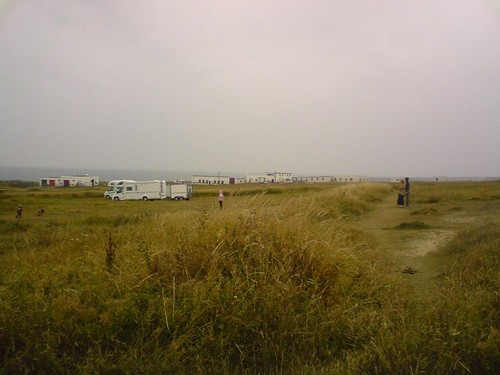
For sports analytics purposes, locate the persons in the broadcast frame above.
[404,177,410,206]
[16,204,23,219]
[218,190,223,209]
[397,179,405,208]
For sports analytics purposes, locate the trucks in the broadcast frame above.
[104,178,193,202]
